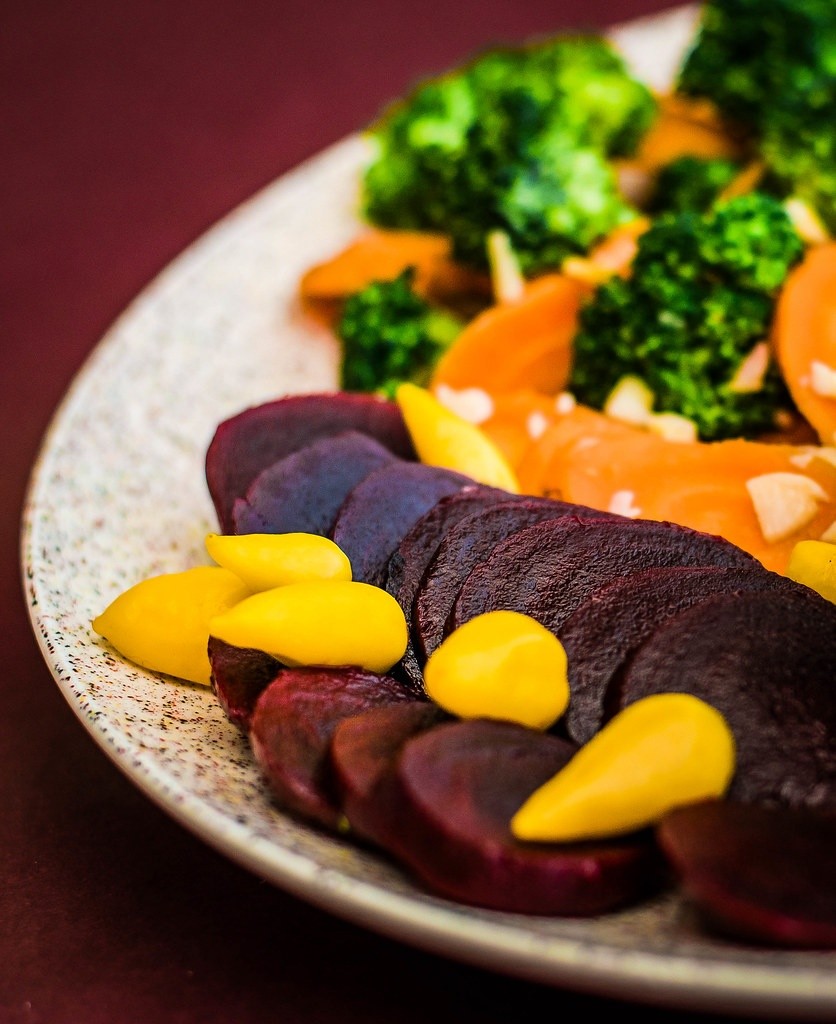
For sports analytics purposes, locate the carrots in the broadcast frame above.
[294,84,835,606]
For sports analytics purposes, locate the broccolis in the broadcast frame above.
[328,0,836,441]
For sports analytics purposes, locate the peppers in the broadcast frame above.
[91,531,736,844]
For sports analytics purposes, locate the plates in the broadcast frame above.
[20,3,836,1019]
